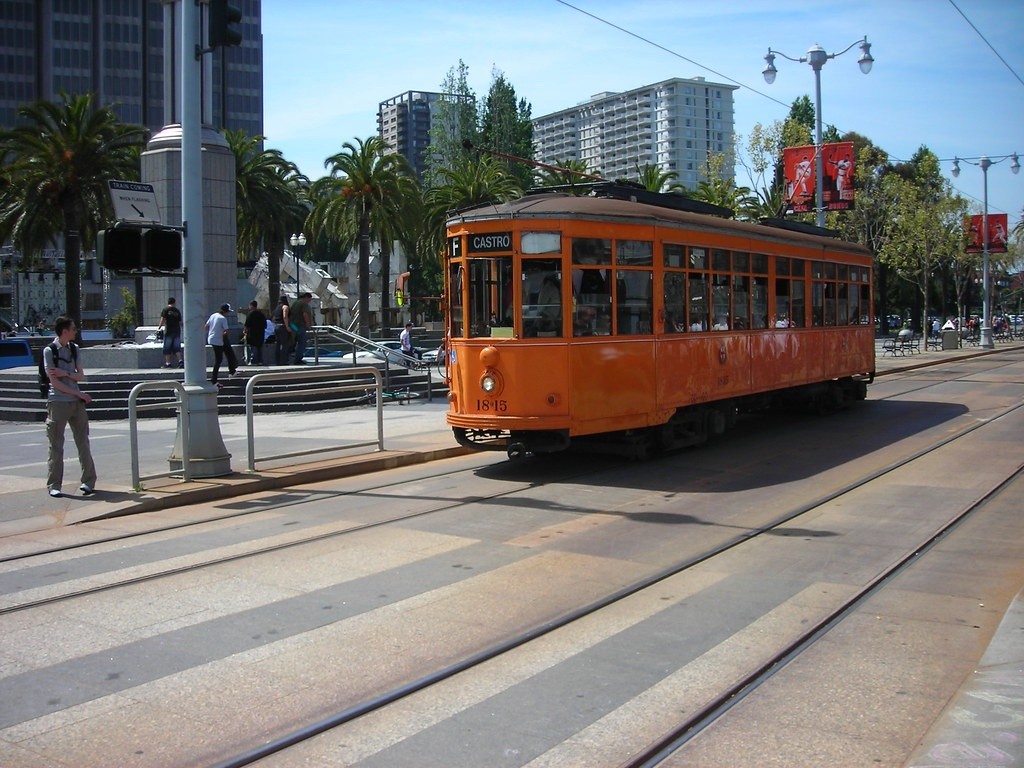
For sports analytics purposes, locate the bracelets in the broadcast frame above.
[65,370,66,377]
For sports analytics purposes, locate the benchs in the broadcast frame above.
[926,332,947,352]
[900,332,924,355]
[965,331,981,346]
[992,327,1024,343]
[882,335,906,357]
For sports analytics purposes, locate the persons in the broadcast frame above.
[400,324,423,367]
[205,303,241,389]
[39,319,47,336]
[828,154,850,198]
[243,301,267,366]
[158,297,184,369]
[992,313,1010,336]
[928,317,939,339]
[795,156,811,195]
[43,317,96,496]
[502,262,561,337]
[274,293,312,365]
[992,221,1007,244]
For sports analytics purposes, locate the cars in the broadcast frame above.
[421,347,446,364]
[302,346,345,358]
[343,340,422,366]
[969,314,1024,325]
[861,314,941,329]
[0,339,35,370]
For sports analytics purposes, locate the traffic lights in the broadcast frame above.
[207,0,243,46]
[143,228,182,272]
[93,223,143,269]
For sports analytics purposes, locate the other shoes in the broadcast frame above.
[294,360,306,364]
[49,488,61,496]
[178,360,184,368]
[212,383,223,388]
[79,482,92,495]
[161,363,171,368]
[229,371,239,378]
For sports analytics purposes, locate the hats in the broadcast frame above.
[221,303,234,312]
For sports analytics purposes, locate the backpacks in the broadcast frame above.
[39,341,77,398]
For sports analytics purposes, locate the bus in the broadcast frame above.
[436,177,876,466]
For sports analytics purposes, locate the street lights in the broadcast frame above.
[290,233,307,298]
[951,151,1022,351]
[761,32,875,223]
